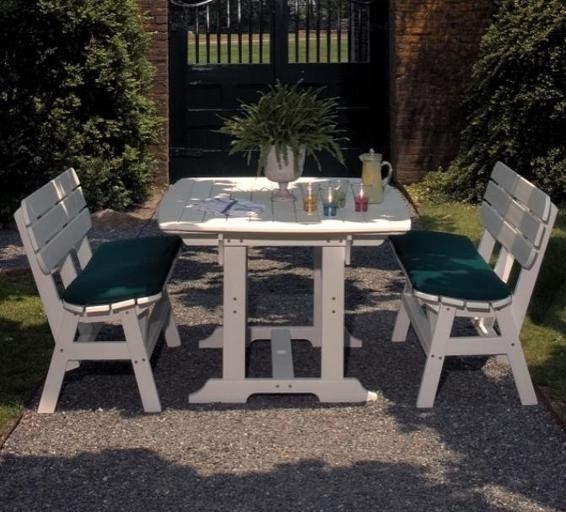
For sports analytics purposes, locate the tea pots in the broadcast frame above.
[359,148,392,204]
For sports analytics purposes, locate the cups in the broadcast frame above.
[296,181,320,217]
[319,182,337,219]
[351,182,373,214]
[331,179,349,208]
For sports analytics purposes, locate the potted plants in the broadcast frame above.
[213,74,354,205]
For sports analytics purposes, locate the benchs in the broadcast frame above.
[13,167,184,418]
[388,160,560,409]
